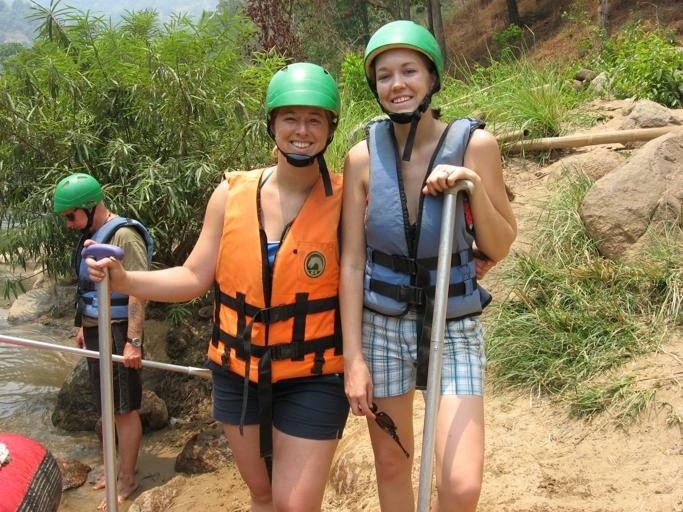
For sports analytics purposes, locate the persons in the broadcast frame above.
[53,172,154,511]
[338,20,518,512]
[83,61,495,512]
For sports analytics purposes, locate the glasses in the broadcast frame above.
[66,208,77,220]
[370,402,411,459]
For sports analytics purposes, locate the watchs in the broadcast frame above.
[127,337,143,347]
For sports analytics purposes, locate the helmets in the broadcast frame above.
[54,172,104,213]
[265,61,341,120]
[364,20,444,93]
